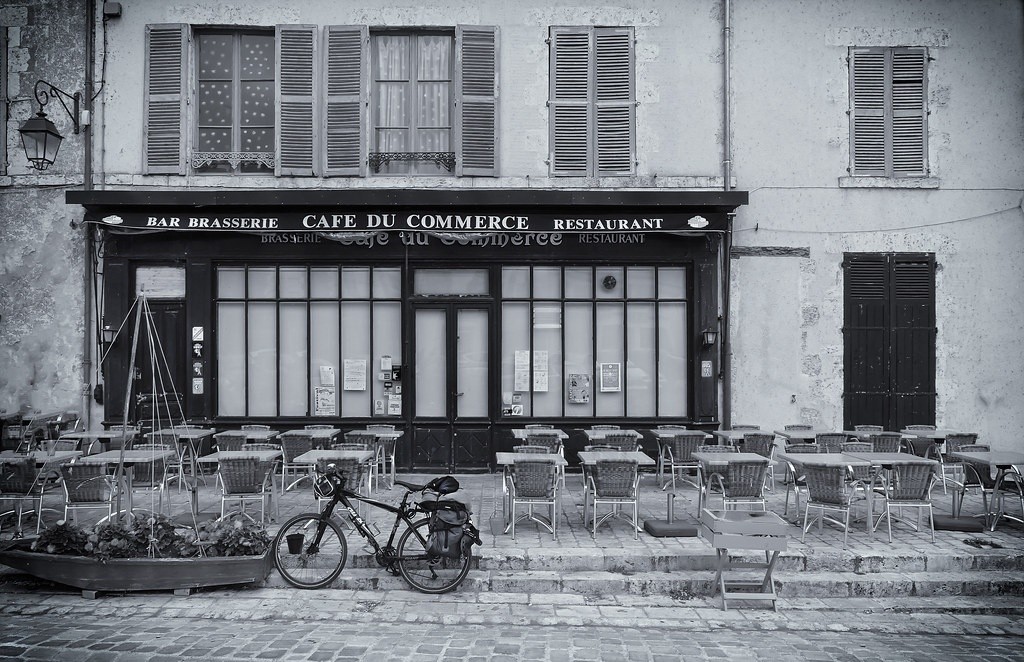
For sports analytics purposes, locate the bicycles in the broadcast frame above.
[272,457,482,596]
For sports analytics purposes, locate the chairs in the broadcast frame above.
[0,412,398,535]
[502,425,1024,550]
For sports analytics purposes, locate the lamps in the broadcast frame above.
[18,79,80,171]
[703,325,719,350]
[98,323,118,357]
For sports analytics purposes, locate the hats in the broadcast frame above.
[464,522,482,546]
[461,536,474,556]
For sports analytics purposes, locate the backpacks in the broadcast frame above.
[425,510,469,557]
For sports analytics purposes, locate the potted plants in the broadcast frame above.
[1,515,279,592]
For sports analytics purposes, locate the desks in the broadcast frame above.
[0,449,84,530]
[583,430,644,440]
[144,429,217,492]
[776,452,871,536]
[213,430,279,439]
[712,431,776,445]
[62,431,140,491]
[649,429,714,489]
[276,429,341,491]
[951,451,1024,529]
[80,449,176,531]
[197,451,282,526]
[577,452,655,533]
[700,508,790,613]
[495,452,568,533]
[841,452,939,529]
[343,430,405,489]
[900,430,978,440]
[691,453,778,511]
[841,431,902,437]
[774,430,834,482]
[293,450,374,530]
[510,429,569,439]
